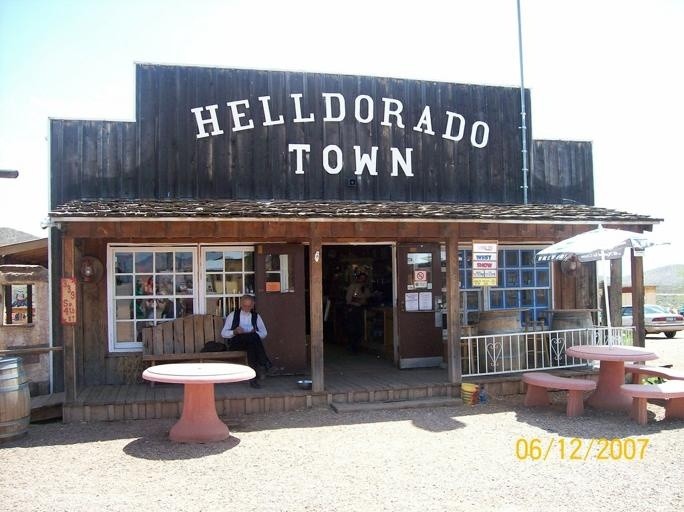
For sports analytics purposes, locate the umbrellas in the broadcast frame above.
[535,222,674,349]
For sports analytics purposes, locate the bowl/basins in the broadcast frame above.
[296,380,313,390]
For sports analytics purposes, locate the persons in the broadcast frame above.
[219,294,280,388]
[143,275,152,322]
[342,271,370,356]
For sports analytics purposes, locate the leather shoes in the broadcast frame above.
[250,379,262,390]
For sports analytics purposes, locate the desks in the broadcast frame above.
[142,362,257,443]
[566,345,659,409]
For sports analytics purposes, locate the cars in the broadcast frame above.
[621,304,684,338]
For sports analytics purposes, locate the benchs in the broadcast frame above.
[141,314,249,388]
[521,363,684,425]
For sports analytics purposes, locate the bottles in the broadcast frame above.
[480,386,488,406]
